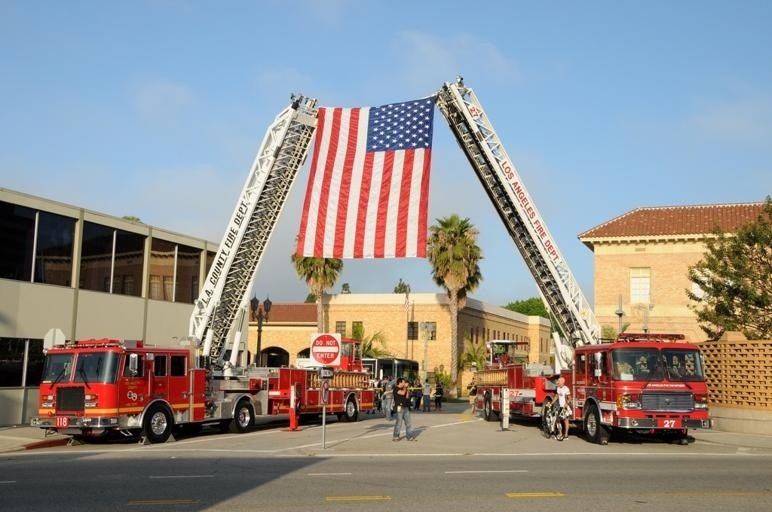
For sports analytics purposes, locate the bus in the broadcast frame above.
[362,359,420,412]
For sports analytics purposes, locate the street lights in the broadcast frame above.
[249,294,272,367]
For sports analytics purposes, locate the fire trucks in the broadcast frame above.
[32,94,378,445]
[436,76,713,445]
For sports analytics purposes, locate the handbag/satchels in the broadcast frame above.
[401,398,414,407]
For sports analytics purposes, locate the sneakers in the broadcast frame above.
[407,436,418,441]
[563,435,569,440]
[391,436,402,441]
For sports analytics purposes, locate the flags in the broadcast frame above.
[296,96,436,259]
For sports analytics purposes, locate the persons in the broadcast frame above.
[546,377,572,441]
[467,382,477,417]
[392,378,415,442]
[372,376,443,421]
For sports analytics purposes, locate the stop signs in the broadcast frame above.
[309,333,342,367]
